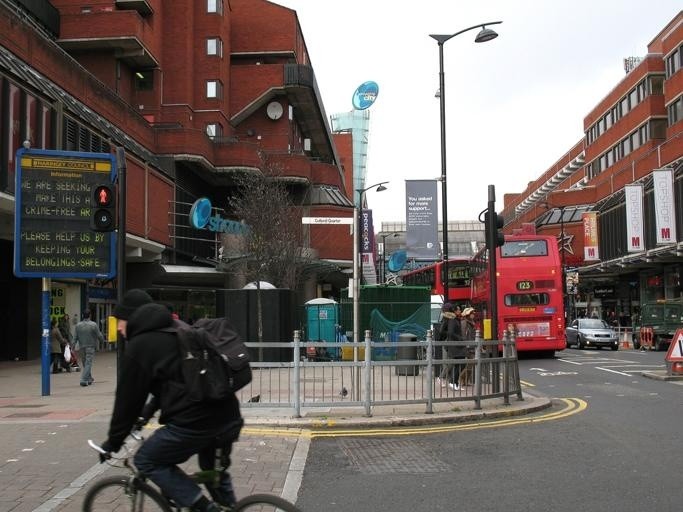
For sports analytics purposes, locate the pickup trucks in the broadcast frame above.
[631,298,682,352]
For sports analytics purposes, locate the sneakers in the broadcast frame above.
[204,501,224,512]
[436,377,446,388]
[458,386,464,391]
[449,382,458,391]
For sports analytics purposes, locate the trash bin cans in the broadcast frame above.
[395,333,419,376]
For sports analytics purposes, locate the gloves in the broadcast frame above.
[134,418,144,430]
[99,438,121,463]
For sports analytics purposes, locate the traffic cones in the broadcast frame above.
[620,329,630,349]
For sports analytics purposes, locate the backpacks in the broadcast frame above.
[155,317,251,402]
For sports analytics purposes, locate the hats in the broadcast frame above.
[111,288,155,322]
[461,308,474,316]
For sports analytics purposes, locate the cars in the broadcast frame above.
[565,318,619,352]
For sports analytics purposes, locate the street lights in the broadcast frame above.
[536,201,569,310]
[426,19,504,307]
[355,181,390,287]
[380,232,406,282]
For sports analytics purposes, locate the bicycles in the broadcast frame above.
[79,418,299,512]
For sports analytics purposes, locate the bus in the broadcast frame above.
[401,257,486,310]
[469,234,566,359]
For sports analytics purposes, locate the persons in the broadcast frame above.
[99,286,245,512]
[50,313,79,373]
[138,300,238,509]
[71,308,104,387]
[436,302,477,392]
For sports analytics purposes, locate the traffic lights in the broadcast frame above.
[88,181,116,234]
[485,210,504,249]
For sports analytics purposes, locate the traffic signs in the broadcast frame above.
[12,145,117,281]
